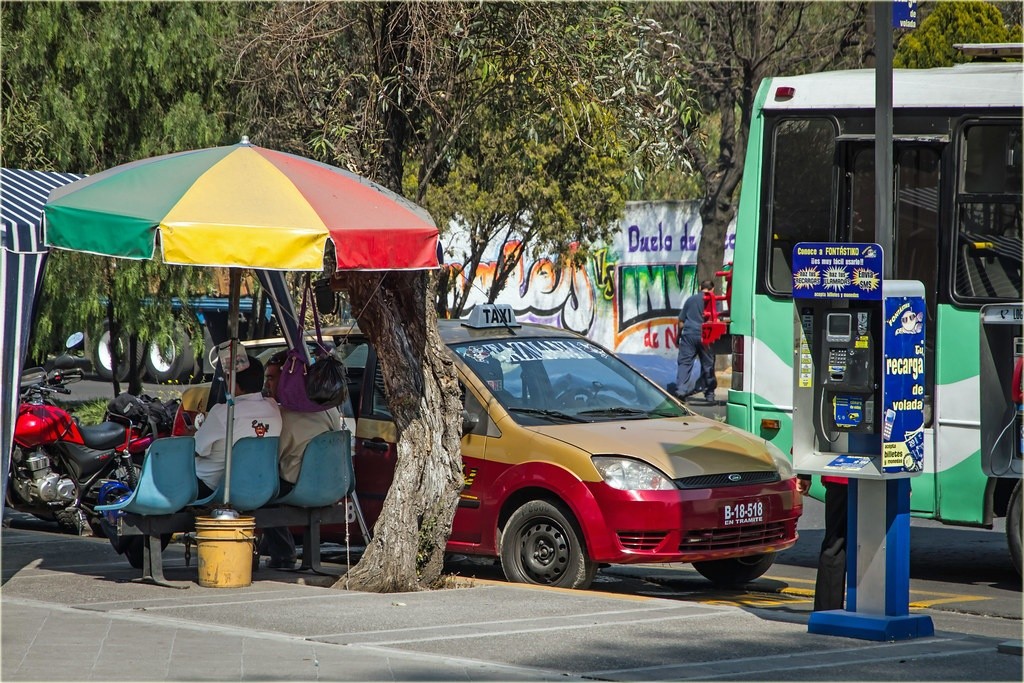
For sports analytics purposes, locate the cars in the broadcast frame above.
[215,302,806,591]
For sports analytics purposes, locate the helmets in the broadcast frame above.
[98,481,133,518]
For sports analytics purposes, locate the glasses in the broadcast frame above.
[267,355,285,366]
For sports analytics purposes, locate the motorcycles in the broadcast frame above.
[6,330,192,542]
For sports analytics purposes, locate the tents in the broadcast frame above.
[0,167,87,513]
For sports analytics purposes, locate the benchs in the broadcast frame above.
[95,430,372,589]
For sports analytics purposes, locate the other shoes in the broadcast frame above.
[704,392,715,401]
[124,547,138,568]
[101,518,123,555]
[252,558,259,571]
[265,554,297,568]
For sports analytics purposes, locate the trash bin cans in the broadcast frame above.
[193,514,256,590]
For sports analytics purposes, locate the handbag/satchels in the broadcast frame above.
[277,346,348,412]
[107,394,181,433]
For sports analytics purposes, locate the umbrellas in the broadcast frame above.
[39,137,442,508]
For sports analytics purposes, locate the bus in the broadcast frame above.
[727,41,1023,587]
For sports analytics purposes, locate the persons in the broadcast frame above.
[99,352,358,572]
[675,280,719,403]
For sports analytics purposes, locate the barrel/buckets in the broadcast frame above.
[195,514,258,589]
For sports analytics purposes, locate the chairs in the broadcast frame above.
[468,355,515,415]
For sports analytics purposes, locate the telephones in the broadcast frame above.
[819,308,875,394]
